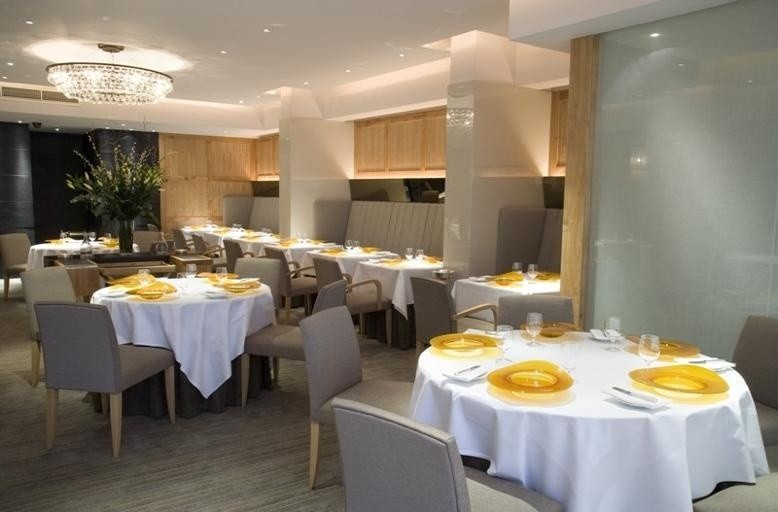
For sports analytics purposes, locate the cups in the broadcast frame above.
[559,340,582,374]
[636,333,664,365]
[232,224,242,233]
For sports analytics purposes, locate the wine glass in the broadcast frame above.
[49,230,119,247]
[525,311,543,348]
[511,261,539,284]
[493,324,514,363]
[601,317,621,353]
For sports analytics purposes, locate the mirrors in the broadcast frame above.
[541,176,564,208]
[250,181,279,197]
[348,178,445,205]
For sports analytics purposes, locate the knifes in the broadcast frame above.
[612,384,657,404]
[454,364,480,375]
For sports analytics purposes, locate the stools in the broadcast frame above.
[692,468,778,511]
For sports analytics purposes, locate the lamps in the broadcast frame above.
[44,43,176,107]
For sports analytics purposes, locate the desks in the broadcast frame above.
[183,223,442,353]
[90,273,272,425]
[29,240,138,267]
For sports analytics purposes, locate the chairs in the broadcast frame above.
[297,304,413,489]
[20,268,77,385]
[329,397,566,511]
[239,279,348,411]
[32,301,176,457]
[131,223,254,273]
[233,258,282,326]
[1,233,33,297]
[410,276,499,361]
[730,313,777,448]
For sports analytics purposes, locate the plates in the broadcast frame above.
[475,371,491,381]
[616,397,644,408]
[470,277,486,282]
[485,362,574,396]
[277,237,323,248]
[712,367,729,373]
[428,332,496,355]
[626,364,730,397]
[318,240,438,267]
[519,319,578,337]
[487,269,557,287]
[626,330,700,359]
[588,333,595,340]
[107,264,265,300]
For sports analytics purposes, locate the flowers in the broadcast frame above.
[63,129,179,255]
